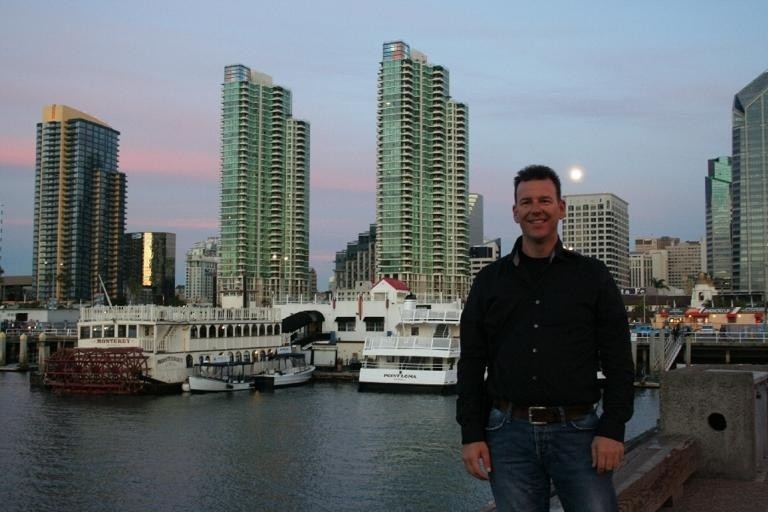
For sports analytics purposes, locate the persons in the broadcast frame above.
[455,165,634,511]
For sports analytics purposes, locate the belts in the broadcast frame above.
[494,399,595,425]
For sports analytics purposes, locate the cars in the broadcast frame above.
[1,303,66,309]
[628,323,719,339]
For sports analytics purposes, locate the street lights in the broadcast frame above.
[270,252,290,304]
[42,260,63,309]
[640,291,647,323]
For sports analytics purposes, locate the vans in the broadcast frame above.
[719,322,767,343]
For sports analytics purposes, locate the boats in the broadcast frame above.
[632,379,659,389]
